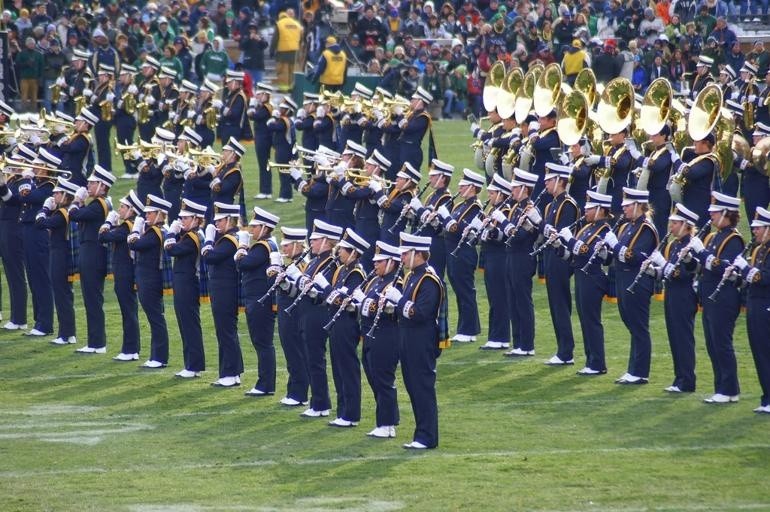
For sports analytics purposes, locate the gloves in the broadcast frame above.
[411,196,421,210]
[158,158,164,166]
[272,107,324,118]
[289,168,301,181]
[286,263,330,289]
[470,121,544,145]
[559,153,569,164]
[401,204,416,217]
[270,251,284,266]
[354,101,402,118]
[55,77,156,105]
[106,210,118,225]
[133,150,142,159]
[437,205,505,231]
[238,231,249,250]
[370,182,383,193]
[630,146,641,159]
[205,224,217,243]
[177,162,188,171]
[338,286,403,304]
[43,197,57,211]
[209,165,216,179]
[518,207,617,260]
[187,110,195,119]
[641,237,747,281]
[583,155,601,167]
[148,95,179,124]
[73,188,87,201]
[671,152,679,163]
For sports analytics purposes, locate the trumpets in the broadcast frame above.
[320,84,411,121]
[114,136,224,167]
[266,141,397,189]
[1,107,75,180]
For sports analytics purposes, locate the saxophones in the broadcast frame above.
[48,64,230,129]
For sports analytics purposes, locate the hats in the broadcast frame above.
[429,158,570,197]
[683,56,770,136]
[279,220,431,262]
[316,140,422,183]
[583,187,770,229]
[0,101,118,193]
[178,69,296,110]
[119,188,278,231]
[72,49,177,82]
[302,81,434,106]
[151,124,245,157]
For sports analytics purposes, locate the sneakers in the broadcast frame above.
[254,194,273,199]
[451,334,606,375]
[617,372,770,413]
[276,196,293,203]
[3,320,139,361]
[142,361,275,396]
[281,396,428,449]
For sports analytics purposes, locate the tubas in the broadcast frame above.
[470,59,770,183]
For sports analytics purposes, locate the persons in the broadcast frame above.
[1,0,770,120]
[1,54,452,451]
[447,109,770,412]
